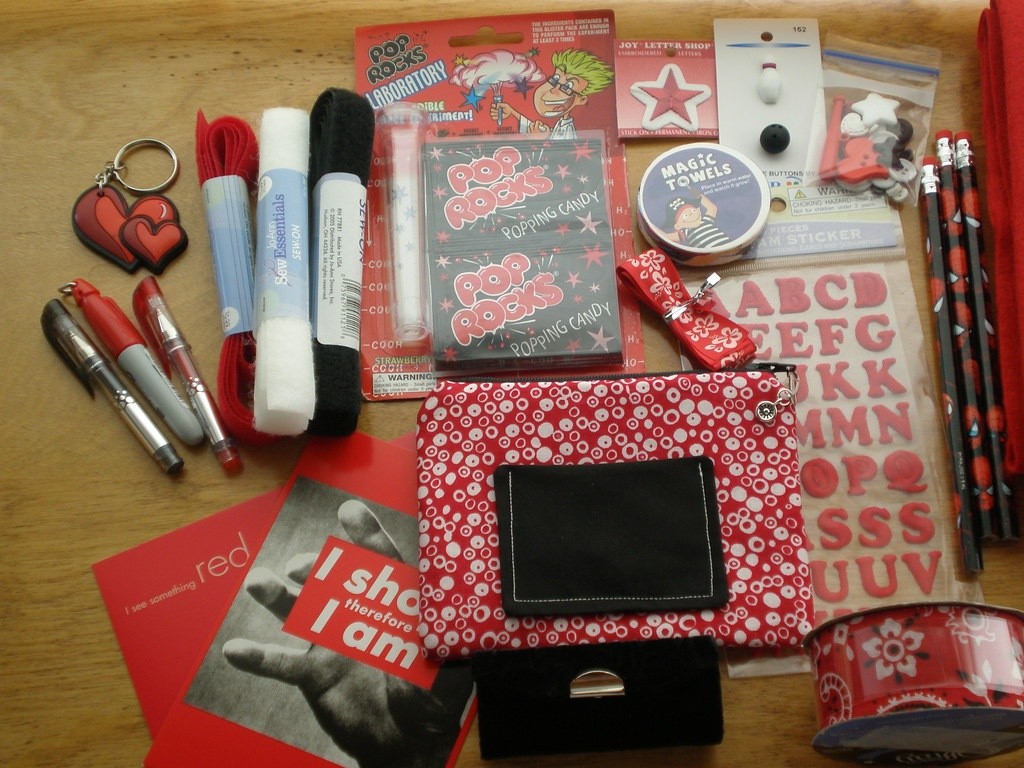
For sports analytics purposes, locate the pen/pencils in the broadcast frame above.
[132,274,243,476]
[920,126,1023,574]
[40,298,185,475]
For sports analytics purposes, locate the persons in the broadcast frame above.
[223,499,478,768]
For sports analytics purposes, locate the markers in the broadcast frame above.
[54,279,206,447]
